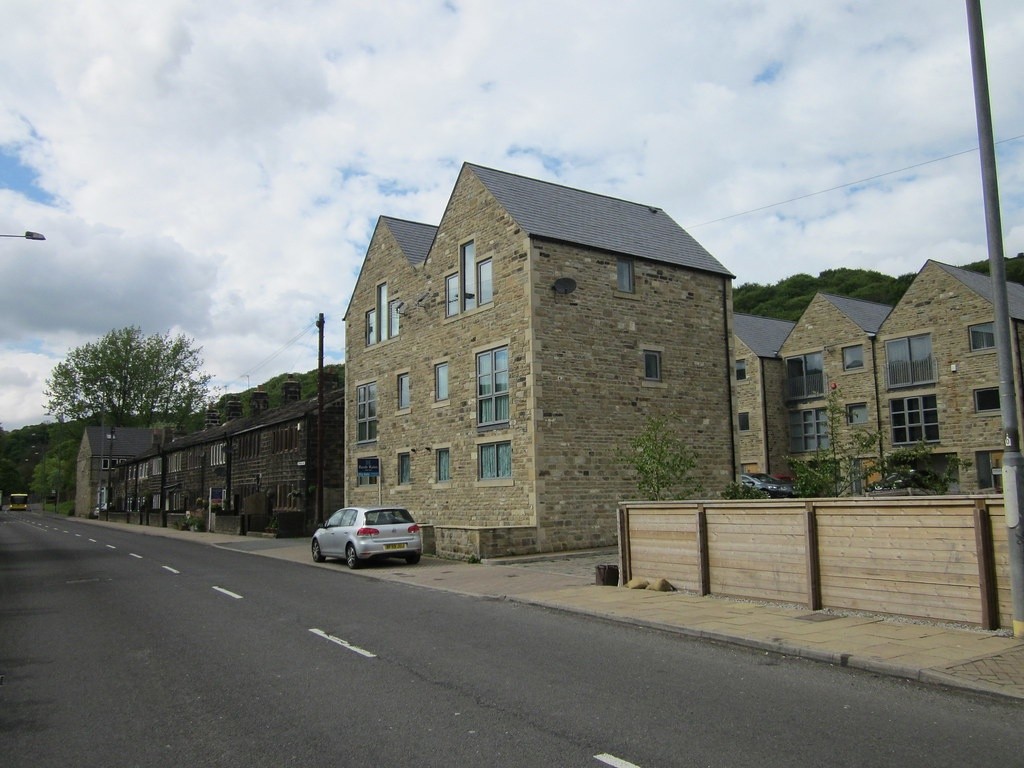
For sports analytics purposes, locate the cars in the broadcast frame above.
[864,469,939,493]
[741,473,800,499]
[93,501,113,518]
[311,506,422,570]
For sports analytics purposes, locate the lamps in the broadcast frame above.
[395,302,407,319]
[416,294,427,309]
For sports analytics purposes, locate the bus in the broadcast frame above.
[8,494,28,511]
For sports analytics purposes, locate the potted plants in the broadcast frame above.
[265,516,279,533]
[182,514,207,533]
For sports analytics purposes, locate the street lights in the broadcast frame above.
[32,432,45,510]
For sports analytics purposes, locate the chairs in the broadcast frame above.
[375,512,389,525]
